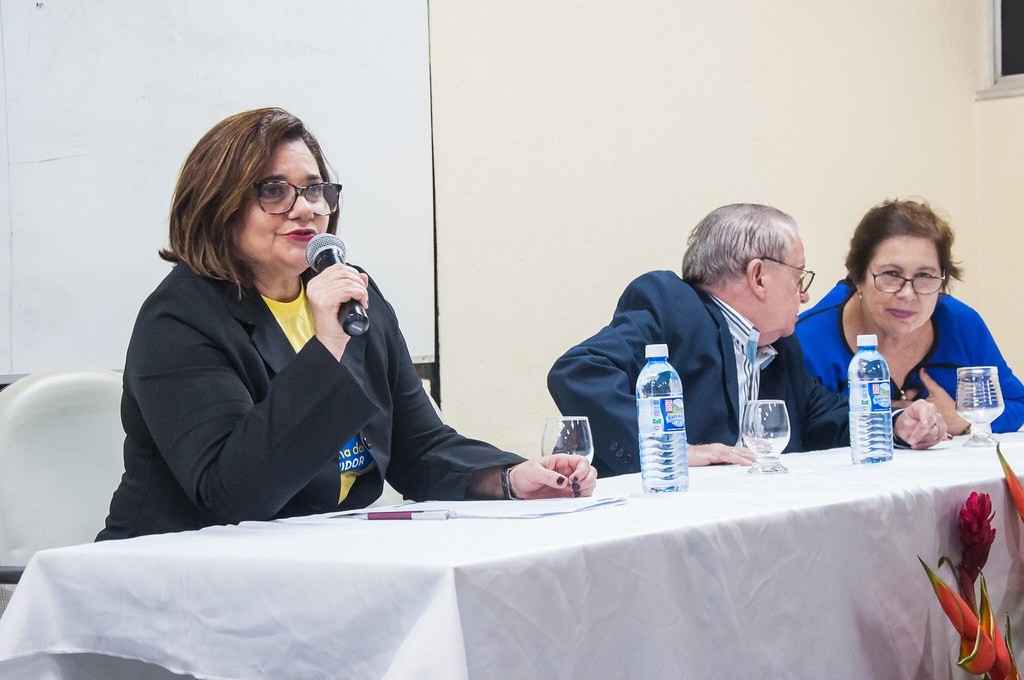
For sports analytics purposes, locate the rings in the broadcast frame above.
[731,449,738,454]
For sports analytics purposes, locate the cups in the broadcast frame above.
[540,415,594,467]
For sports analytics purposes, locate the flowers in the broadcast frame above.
[914,442,1024,680]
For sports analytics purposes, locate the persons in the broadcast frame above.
[547,203,947,481]
[94,107,597,542]
[794,197,1024,436]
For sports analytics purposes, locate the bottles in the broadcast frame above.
[847,335,894,465]
[635,343,690,497]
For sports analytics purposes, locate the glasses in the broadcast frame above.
[253,180,342,216]
[761,256,815,292]
[865,264,946,295]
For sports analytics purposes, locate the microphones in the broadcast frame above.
[304,234,371,337]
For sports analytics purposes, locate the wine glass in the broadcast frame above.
[742,399,791,475]
[954,366,1005,448]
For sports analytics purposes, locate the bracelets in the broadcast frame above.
[501,464,521,500]
[959,423,971,436]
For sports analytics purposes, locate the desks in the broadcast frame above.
[0,432,1024,680]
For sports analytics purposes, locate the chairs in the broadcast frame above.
[0,371,129,604]
